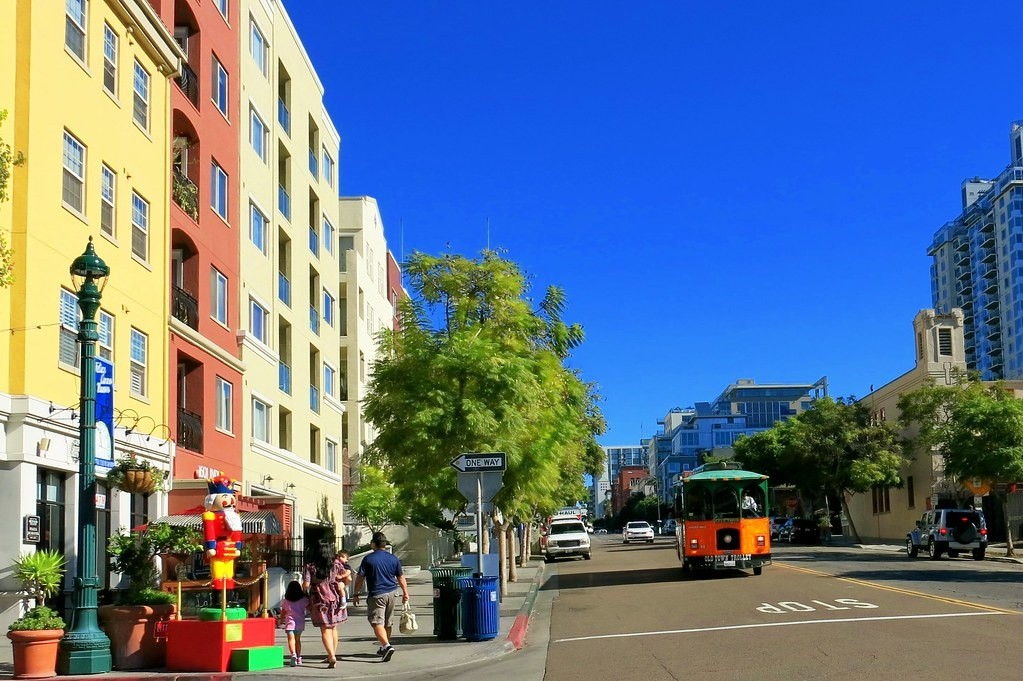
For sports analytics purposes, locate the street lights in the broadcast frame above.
[56,233,112,676]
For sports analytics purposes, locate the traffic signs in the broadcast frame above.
[450,451,507,473]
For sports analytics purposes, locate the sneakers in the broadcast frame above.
[376,646,384,656]
[297,657,303,666]
[382,645,394,662]
[290,654,297,666]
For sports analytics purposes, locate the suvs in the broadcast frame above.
[905,508,988,561]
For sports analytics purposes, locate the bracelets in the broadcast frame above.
[353,594,359,597]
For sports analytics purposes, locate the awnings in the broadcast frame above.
[154,511,281,535]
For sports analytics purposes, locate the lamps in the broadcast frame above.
[264,474,274,483]
[286,482,296,489]
[49,402,171,446]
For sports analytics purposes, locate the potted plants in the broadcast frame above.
[6,548,71,679]
[98,522,204,670]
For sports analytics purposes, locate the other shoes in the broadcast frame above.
[338,602,347,609]
[328,657,336,668]
[322,658,330,663]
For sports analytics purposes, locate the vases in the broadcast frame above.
[117,469,157,493]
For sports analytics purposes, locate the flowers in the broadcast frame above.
[105,450,166,498]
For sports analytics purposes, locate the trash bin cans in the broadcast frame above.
[429,566,474,641]
[458,576,499,642]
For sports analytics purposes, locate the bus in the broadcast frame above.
[671,461,773,575]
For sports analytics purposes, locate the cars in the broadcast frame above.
[622,520,659,544]
[661,518,676,536]
[542,520,592,564]
[769,515,791,542]
[778,516,826,547]
[543,505,621,534]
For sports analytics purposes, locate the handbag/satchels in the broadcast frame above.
[399,601,418,634]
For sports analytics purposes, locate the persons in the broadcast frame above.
[736,488,758,511]
[278,581,311,666]
[336,549,351,610]
[302,539,352,669]
[203,476,243,590]
[353,532,409,663]
